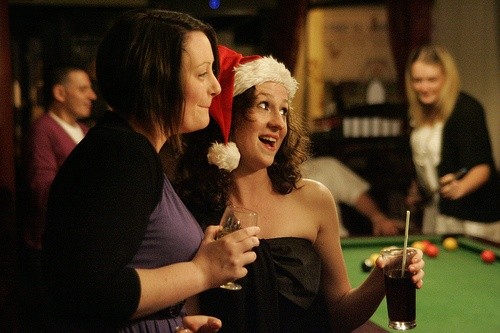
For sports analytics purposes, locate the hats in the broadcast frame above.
[202,43,299,174]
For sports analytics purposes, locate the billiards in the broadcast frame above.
[480,249,496,263]
[411,240,424,254]
[362,258,375,272]
[369,253,380,266]
[422,239,432,253]
[442,237,459,252]
[426,244,440,257]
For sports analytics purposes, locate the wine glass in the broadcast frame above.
[218,204,257,289]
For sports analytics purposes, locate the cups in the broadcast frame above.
[381,247,418,330]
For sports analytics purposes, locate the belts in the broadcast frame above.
[138,298,188,319]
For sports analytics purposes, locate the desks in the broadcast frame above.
[341,232,500,333]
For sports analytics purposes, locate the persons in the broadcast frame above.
[389,45,500,244]
[23,62,97,251]
[297,156,409,240]
[29,8,261,332]
[159,45,421,332]
